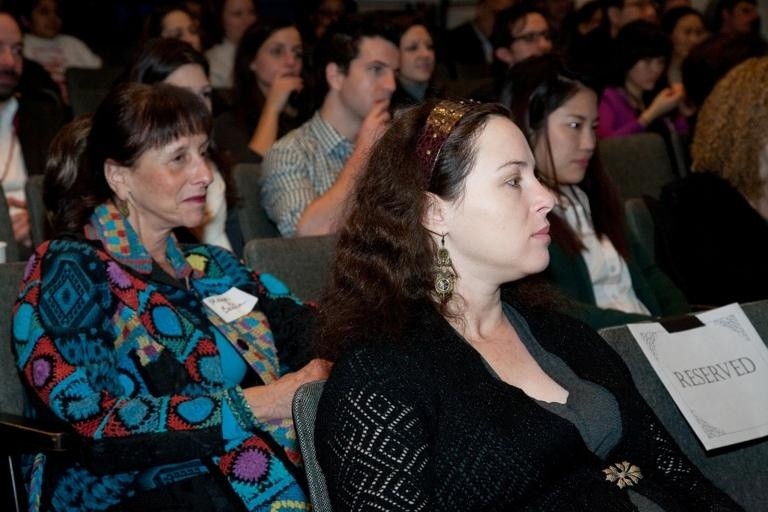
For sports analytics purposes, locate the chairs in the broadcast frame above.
[0,52,766,512]
